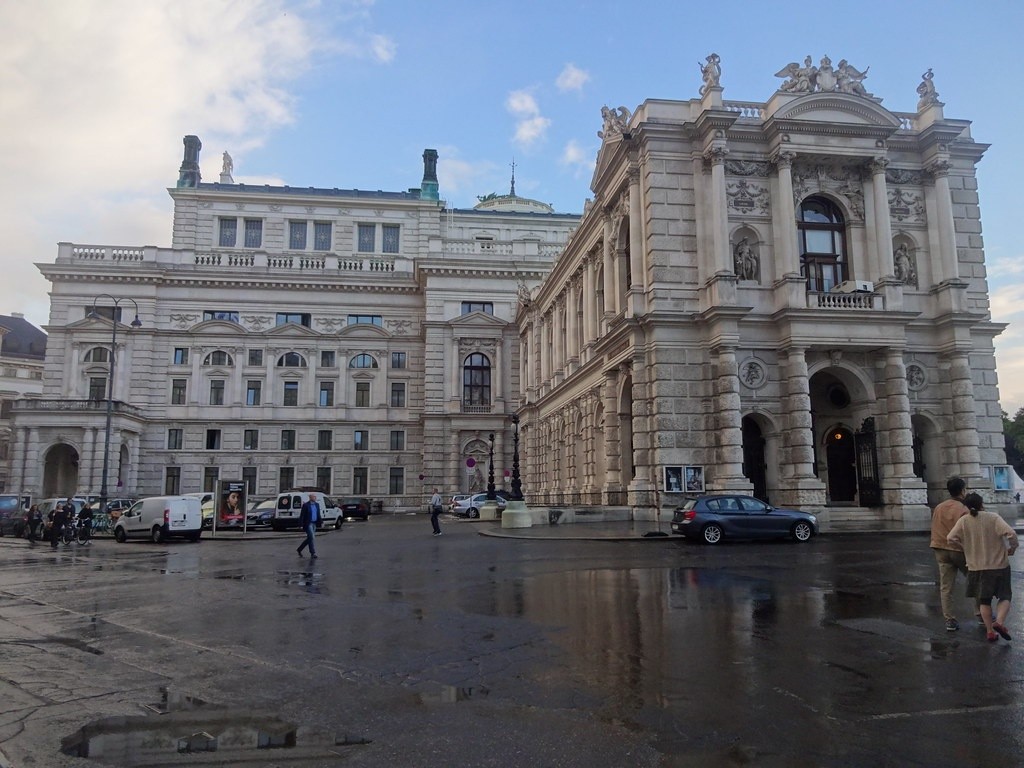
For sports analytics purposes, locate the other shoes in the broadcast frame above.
[987,633,999,641]
[311,555,317,558]
[51,542,59,547]
[946,620,959,631]
[993,622,1011,641]
[297,550,303,556]
[88,540,92,544]
[432,531,441,536]
[978,614,997,625]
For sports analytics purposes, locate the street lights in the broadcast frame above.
[87,293,143,515]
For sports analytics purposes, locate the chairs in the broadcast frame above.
[720,500,729,509]
[731,501,738,509]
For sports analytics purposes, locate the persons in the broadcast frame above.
[27,505,42,543]
[947,494,1019,642]
[832,59,883,105]
[735,236,758,281]
[78,503,93,545]
[220,491,242,525]
[469,466,484,490]
[895,243,915,285]
[296,493,323,558]
[48,503,64,547]
[595,105,632,138]
[63,498,76,533]
[697,52,722,94]
[222,149,233,173]
[776,55,818,93]
[929,476,995,630]
[918,66,943,109]
[427,488,442,536]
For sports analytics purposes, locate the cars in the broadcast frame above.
[453,494,508,518]
[25,498,90,541]
[338,497,372,521]
[0,496,28,537]
[673,492,819,544]
[246,498,276,527]
[91,498,137,519]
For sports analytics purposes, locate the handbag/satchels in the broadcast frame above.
[47,521,53,528]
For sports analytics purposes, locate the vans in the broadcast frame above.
[275,490,344,531]
[113,496,204,544]
[449,495,471,515]
[182,493,214,528]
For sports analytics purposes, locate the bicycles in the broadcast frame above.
[92,515,117,535]
[61,517,92,545]
